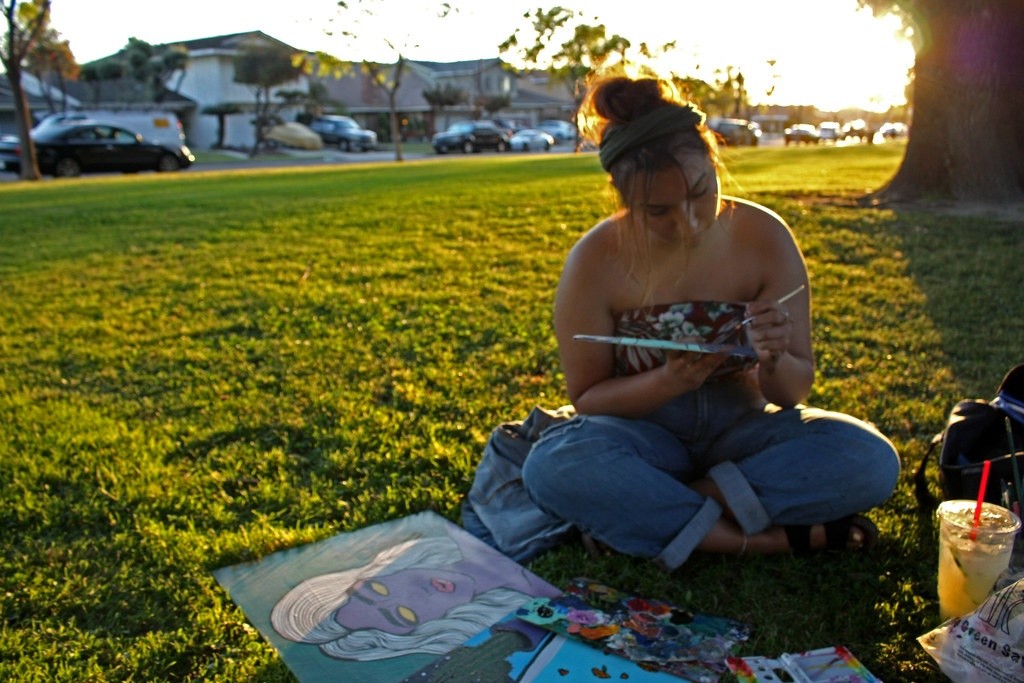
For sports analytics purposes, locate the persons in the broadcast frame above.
[521,75,901,573]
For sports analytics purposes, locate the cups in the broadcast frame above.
[936,498,1022,626]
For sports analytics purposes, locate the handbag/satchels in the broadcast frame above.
[915,578,1024,683]
[909,395,1023,535]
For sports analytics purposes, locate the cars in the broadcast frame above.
[311,115,378,153]
[498,119,531,132]
[0,120,194,180]
[714,120,760,146]
[784,124,821,146]
[507,129,555,152]
[431,120,511,155]
[538,120,576,141]
[818,119,910,144]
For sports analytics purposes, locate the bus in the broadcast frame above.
[32,110,187,153]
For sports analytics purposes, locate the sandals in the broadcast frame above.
[581,531,611,560]
[780,513,881,558]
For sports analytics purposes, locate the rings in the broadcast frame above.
[783,311,789,321]
